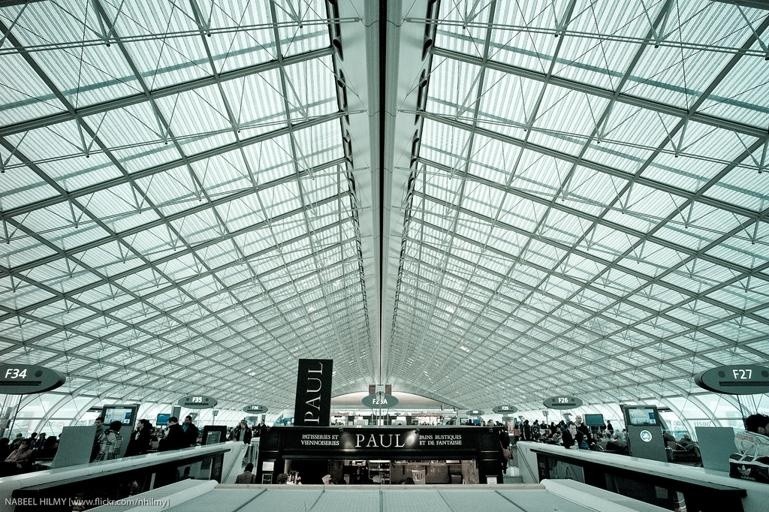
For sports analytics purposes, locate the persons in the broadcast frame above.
[2,416,267,512]
[334,418,338,425]
[466,415,627,474]
[736,414,769,455]
[565,466,574,479]
[277,473,289,484]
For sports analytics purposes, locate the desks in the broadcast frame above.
[516,440,769,512]
[1,439,231,512]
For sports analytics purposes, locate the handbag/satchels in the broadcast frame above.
[728,452,769,484]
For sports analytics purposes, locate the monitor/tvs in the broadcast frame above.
[157,414,168,422]
[628,408,657,424]
[104,409,132,424]
[585,414,603,425]
